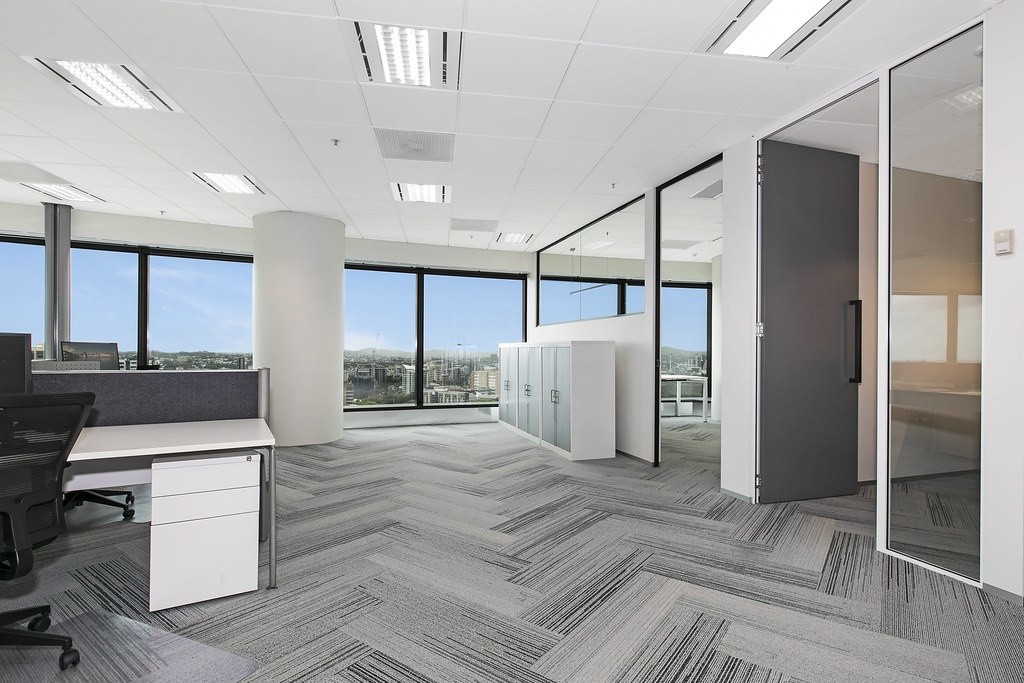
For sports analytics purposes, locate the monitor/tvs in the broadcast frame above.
[61,340,120,369]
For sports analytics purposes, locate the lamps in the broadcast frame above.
[373,23,433,88]
[721,0,834,60]
[403,182,438,204]
[203,171,256,195]
[57,59,159,112]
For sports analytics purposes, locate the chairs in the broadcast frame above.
[0,389,98,674]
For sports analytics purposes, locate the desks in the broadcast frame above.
[0,366,279,618]
